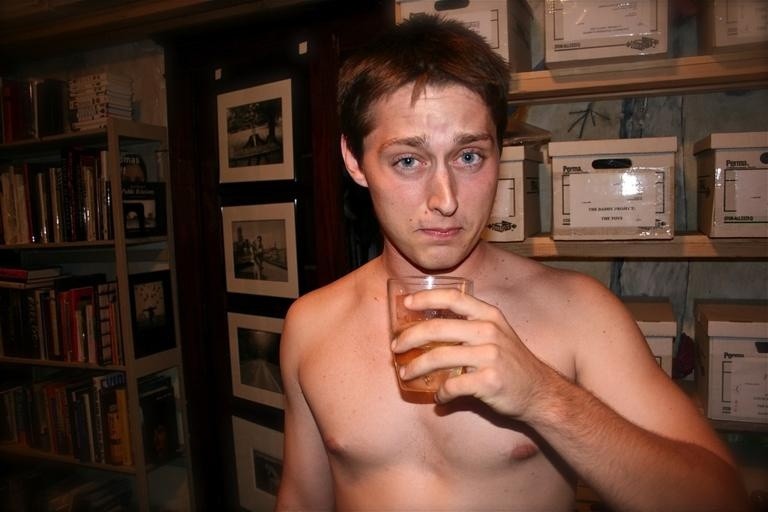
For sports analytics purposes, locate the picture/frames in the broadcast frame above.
[222,308,303,414]
[214,195,305,302]
[226,413,294,510]
[213,72,301,193]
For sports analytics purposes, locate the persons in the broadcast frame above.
[273,11,750,512]
[247,235,266,281]
[241,126,266,166]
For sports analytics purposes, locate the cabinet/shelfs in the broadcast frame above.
[1,70,197,512]
[395,0,768,511]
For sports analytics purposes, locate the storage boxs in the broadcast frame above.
[548,135,678,241]
[693,298,768,425]
[401,0,532,72]
[616,294,677,379]
[692,129,768,239]
[545,1,675,66]
[480,146,544,243]
[696,1,766,55]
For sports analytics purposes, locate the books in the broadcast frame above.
[1,370,176,511]
[1,265,164,366]
[1,152,156,245]
[2,71,135,142]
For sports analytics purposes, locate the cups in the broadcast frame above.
[386,275,472,406]
